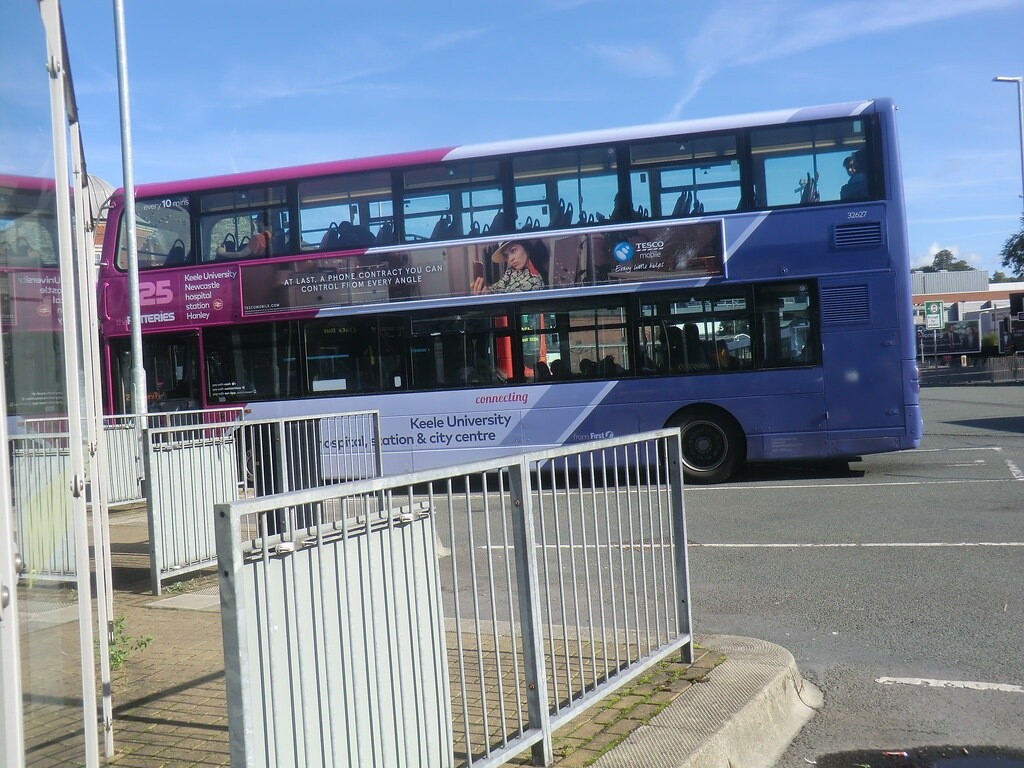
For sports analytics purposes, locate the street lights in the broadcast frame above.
[992,76,1023,219]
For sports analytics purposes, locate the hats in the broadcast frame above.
[491,240,511,263]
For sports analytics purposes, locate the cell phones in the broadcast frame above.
[472,261,484,289]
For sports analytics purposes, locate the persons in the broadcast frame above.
[942,324,960,347]
[471,241,545,296]
[839,149,873,203]
[215,211,274,261]
[334,221,376,248]
[961,327,978,351]
[579,359,598,381]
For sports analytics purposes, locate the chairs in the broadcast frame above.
[165,173,823,266]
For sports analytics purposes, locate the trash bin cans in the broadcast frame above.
[257,421,323,539]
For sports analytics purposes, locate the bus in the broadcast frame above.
[0,175,86,477]
[93,95,925,487]
